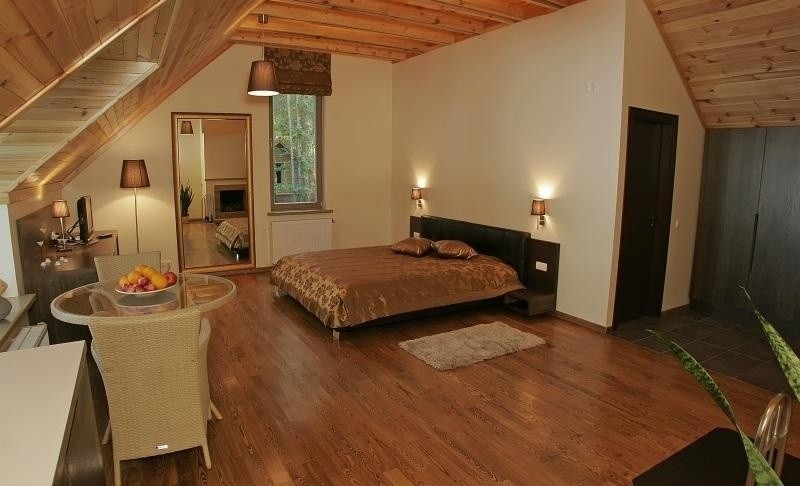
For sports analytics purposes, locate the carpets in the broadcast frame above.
[398,320,546,372]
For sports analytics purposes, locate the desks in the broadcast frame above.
[631,426,800,486]
[49,270,239,446]
[16,203,120,365]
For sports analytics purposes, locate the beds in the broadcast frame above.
[269,214,533,342]
[214,215,250,262]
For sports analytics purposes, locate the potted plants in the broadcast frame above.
[178,179,194,223]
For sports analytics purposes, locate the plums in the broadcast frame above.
[121,277,155,293]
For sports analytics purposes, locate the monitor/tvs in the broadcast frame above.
[77,195,98,245]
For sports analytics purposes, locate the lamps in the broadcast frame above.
[50,197,69,252]
[245,13,278,98]
[118,157,151,253]
[530,197,546,226]
[179,117,194,135]
[410,186,422,208]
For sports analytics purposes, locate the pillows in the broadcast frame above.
[0,278,8,295]
[431,239,479,261]
[0,295,13,322]
[391,236,434,258]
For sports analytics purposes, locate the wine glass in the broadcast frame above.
[35,228,69,256]
[39,254,69,270]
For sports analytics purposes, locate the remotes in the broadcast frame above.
[97,233,113,238]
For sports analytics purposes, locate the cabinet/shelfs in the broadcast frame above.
[0,339,108,486]
[1,293,38,352]
[504,287,555,317]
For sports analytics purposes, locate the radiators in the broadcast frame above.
[266,216,333,266]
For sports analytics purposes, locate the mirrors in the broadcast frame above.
[170,110,257,275]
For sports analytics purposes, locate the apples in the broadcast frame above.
[164,272,177,285]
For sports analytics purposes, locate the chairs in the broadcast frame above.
[85,307,213,485]
[88,251,174,312]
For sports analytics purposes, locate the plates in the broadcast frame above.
[114,283,178,296]
[114,292,177,308]
